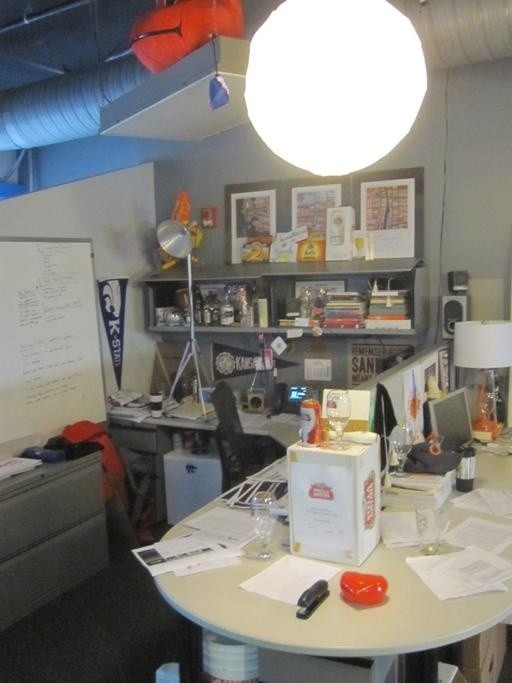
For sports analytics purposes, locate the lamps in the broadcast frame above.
[157,217,212,421]
[451,319,512,422]
[245,0,429,179]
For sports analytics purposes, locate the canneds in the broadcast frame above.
[299,399,321,447]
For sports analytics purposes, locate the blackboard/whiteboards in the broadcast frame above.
[0,237,109,459]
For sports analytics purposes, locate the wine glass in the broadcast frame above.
[250,490,279,560]
[391,422,414,478]
[326,389,350,450]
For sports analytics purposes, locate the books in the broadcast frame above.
[277,318,322,327]
[322,290,414,328]
[109,391,178,422]
[169,402,214,419]
[381,471,457,510]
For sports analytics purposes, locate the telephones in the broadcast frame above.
[273,383,313,414]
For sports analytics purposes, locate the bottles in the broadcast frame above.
[298,285,327,318]
[156,284,268,329]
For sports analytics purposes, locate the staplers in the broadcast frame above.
[296,580,329,619]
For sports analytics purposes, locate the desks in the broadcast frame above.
[109,402,299,521]
[151,427,512,683]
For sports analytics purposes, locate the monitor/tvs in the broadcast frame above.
[423,387,475,457]
[375,346,415,373]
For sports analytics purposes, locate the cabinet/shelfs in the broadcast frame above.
[140,259,428,336]
[0,448,112,635]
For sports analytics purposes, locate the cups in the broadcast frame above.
[171,430,208,452]
[149,393,163,418]
[415,504,441,555]
[456,447,476,492]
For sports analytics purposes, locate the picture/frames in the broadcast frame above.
[225,168,423,263]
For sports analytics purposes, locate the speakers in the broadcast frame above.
[246,390,265,412]
[448,270,468,295]
[441,296,468,339]
